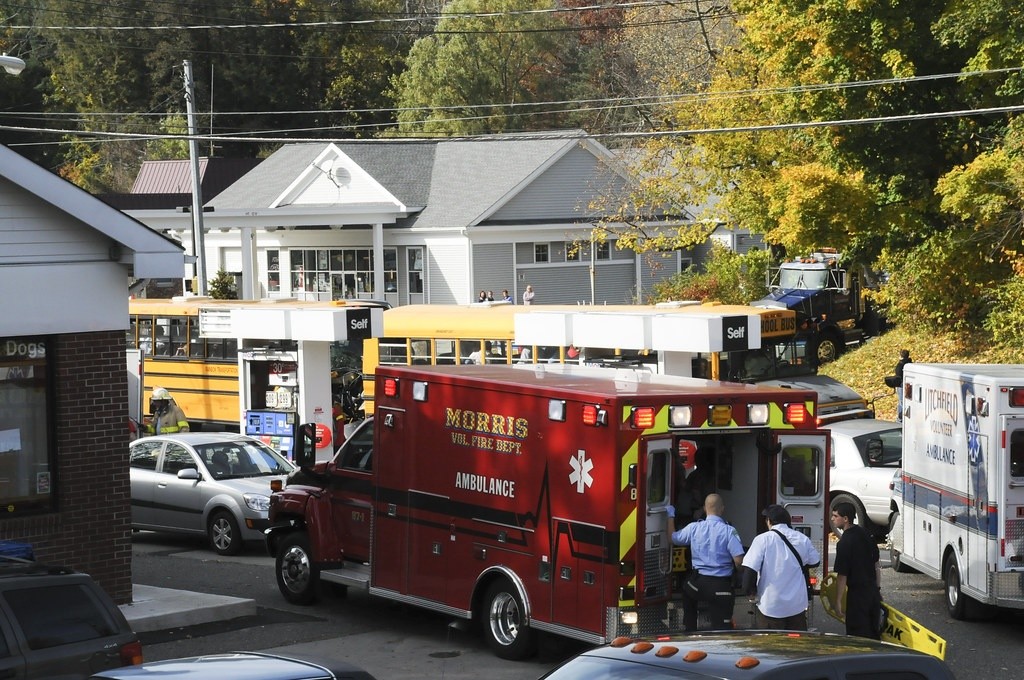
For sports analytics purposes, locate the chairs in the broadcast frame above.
[210,450,230,474]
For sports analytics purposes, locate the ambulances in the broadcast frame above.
[890,363,1024,619]
[261,364,833,660]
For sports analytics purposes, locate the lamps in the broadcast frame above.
[738,237,745,245]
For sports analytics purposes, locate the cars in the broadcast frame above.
[533,629,956,680]
[127,429,301,556]
[86,649,376,680]
[813,417,903,539]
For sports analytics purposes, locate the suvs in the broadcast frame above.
[0,552,143,680]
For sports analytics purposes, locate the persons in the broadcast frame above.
[208,343,221,358]
[501,289,514,304]
[479,291,494,303]
[664,494,745,632]
[138,388,189,436]
[127,319,196,355]
[895,350,913,422]
[523,285,535,305]
[464,341,614,364]
[741,505,820,631]
[831,502,882,641]
[675,446,743,589]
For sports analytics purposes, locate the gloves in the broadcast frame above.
[138,424,147,432]
[664,506,675,517]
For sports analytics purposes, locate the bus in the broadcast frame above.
[382,303,900,425]
[127,297,391,433]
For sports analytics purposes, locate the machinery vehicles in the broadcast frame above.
[749,244,897,367]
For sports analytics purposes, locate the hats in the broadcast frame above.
[762,505,791,525]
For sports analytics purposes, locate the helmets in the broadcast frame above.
[151,388,172,400]
